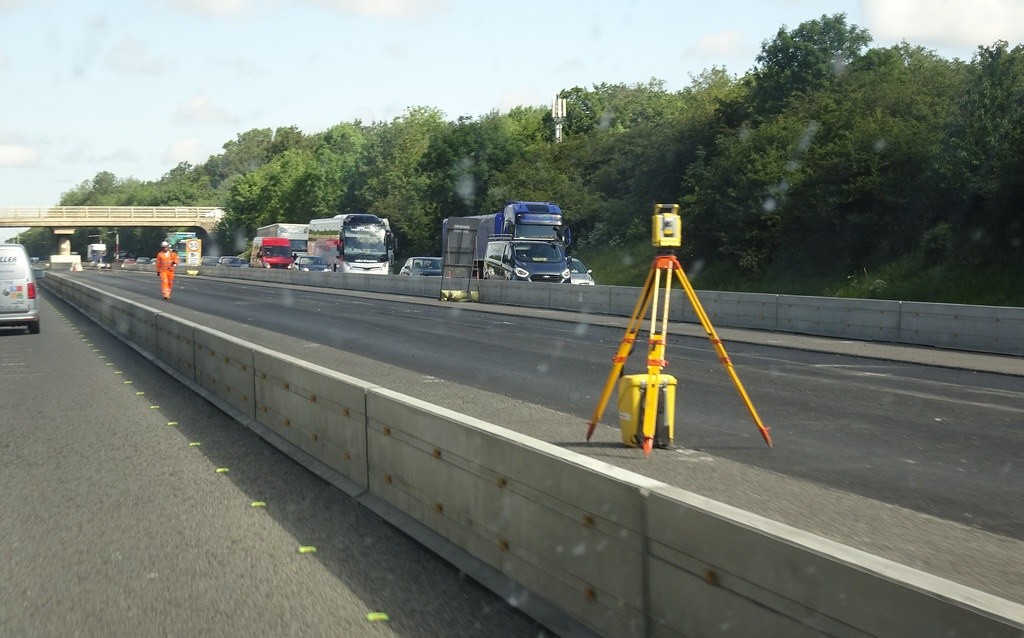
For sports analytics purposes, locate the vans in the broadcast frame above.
[249,238,295,271]
[0,243,39,334]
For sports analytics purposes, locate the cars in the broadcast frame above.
[563,257,594,287]
[399,256,445,280]
[136,255,151,267]
[120,257,136,270]
[290,255,328,273]
[237,258,249,268]
[215,255,241,270]
[202,256,220,268]
[151,258,159,265]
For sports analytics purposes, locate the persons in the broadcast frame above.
[154,240,179,302]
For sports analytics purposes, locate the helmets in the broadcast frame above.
[160,241,168,247]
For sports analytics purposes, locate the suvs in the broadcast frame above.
[476,238,572,284]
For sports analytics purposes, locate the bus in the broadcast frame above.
[307,212,399,278]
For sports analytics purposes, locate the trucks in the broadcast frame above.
[165,232,197,264]
[437,201,572,282]
[254,223,309,258]
[86,243,107,263]
